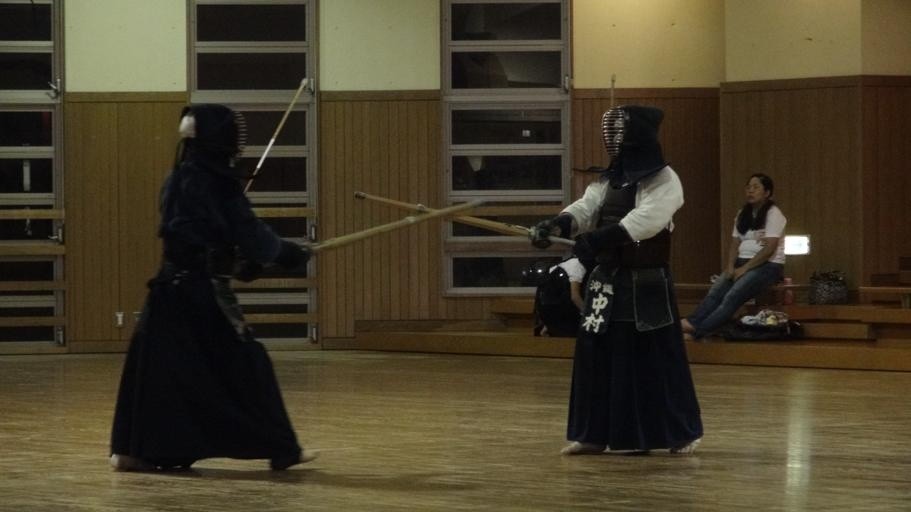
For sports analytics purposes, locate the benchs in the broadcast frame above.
[670,283,911,340]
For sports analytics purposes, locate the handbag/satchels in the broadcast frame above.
[810,270,847,305]
[727,313,801,341]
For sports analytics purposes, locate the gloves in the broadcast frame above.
[575,224,626,262]
[282,237,308,266]
[532,213,571,248]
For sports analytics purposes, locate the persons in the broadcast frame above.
[108,101,322,472]
[680,173,789,341]
[532,256,594,338]
[525,104,706,457]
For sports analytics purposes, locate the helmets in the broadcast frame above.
[178,105,249,171]
[602,104,665,191]
